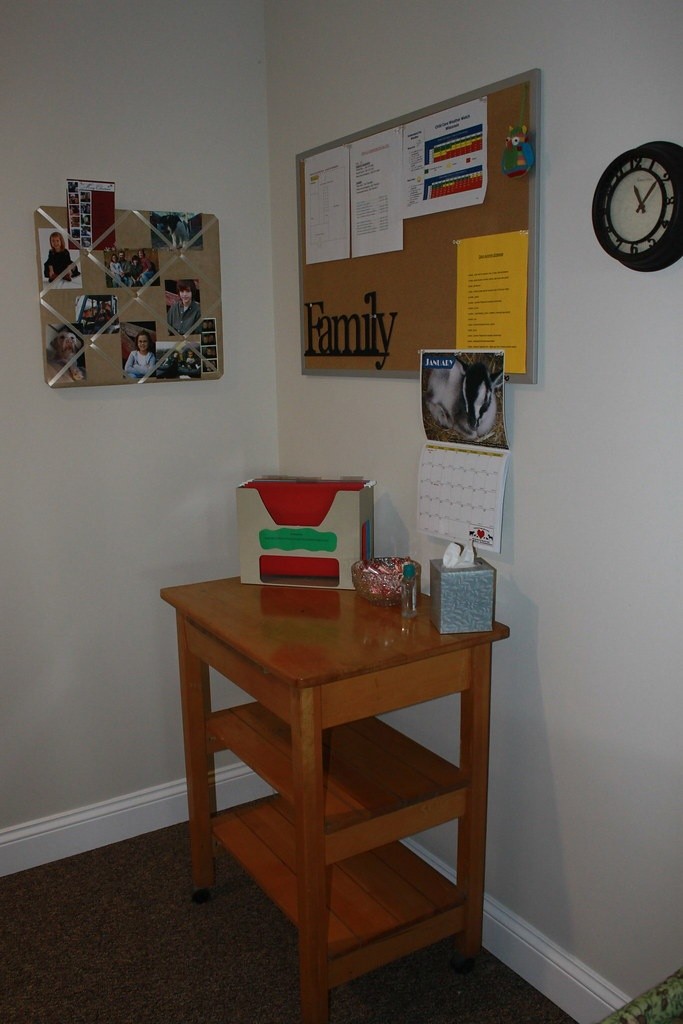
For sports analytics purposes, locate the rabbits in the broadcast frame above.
[426,357,503,442]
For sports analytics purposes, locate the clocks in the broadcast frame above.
[591,141,682,273]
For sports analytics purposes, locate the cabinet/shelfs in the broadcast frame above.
[159,576,511,1024]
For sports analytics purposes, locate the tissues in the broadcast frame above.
[430,539,496,635]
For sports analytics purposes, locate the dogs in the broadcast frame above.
[48,332,83,380]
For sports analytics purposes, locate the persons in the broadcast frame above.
[68,182,91,248]
[201,320,217,371]
[168,280,202,334]
[44,232,81,282]
[162,349,200,372]
[125,331,155,378]
[110,249,157,287]
[89,302,116,334]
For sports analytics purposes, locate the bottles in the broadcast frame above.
[400,562,417,618]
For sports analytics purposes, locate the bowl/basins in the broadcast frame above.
[350,555,422,609]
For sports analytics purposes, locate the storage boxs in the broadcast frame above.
[235,474,375,591]
